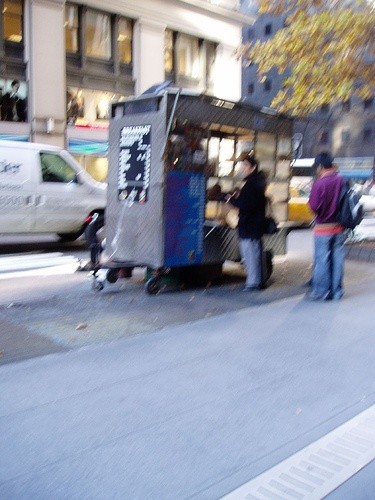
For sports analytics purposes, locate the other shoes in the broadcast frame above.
[240,280,266,292]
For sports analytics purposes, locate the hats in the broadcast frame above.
[312,153,332,168]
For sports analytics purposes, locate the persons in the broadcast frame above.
[228,158,268,291]
[309,151,347,302]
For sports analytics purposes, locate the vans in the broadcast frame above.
[1,139,109,244]
[288,158,318,197]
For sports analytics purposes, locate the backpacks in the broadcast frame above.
[336,181,367,230]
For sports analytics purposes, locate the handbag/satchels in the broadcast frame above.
[261,217,279,234]
